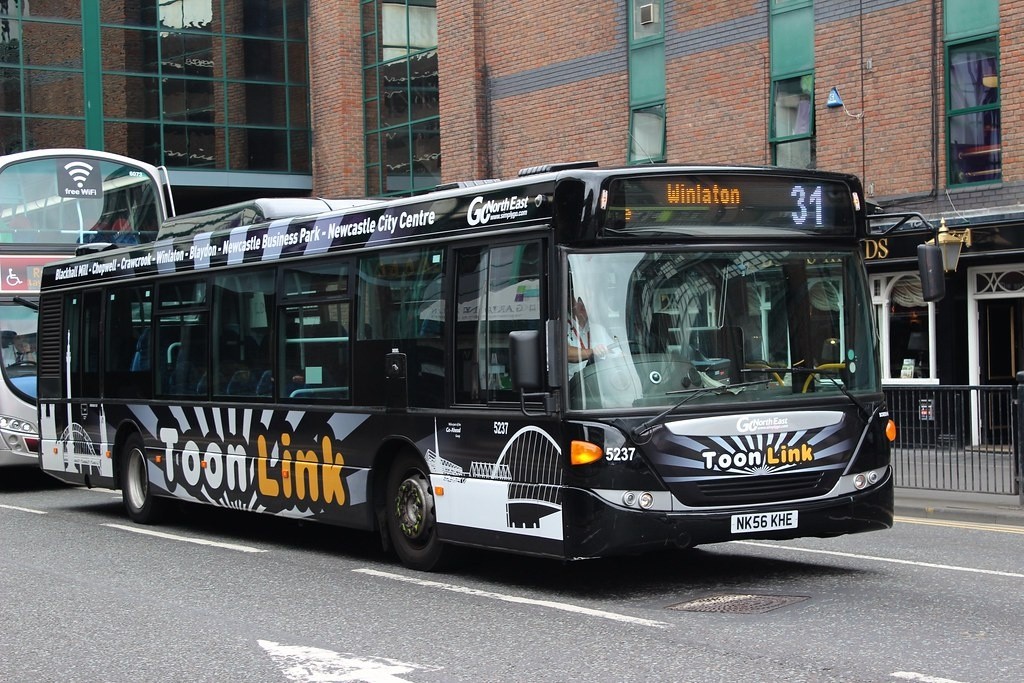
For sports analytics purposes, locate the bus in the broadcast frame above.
[35,164,946,571]
[0,148,177,465]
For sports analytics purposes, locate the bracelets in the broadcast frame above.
[26,351,31,354]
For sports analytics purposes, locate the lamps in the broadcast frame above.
[923,215,974,272]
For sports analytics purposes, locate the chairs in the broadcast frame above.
[10,211,138,244]
[159,345,276,399]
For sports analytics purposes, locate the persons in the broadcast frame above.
[558,288,622,379]
[1,328,37,369]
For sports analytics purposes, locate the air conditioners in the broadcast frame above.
[640,3,659,26]
